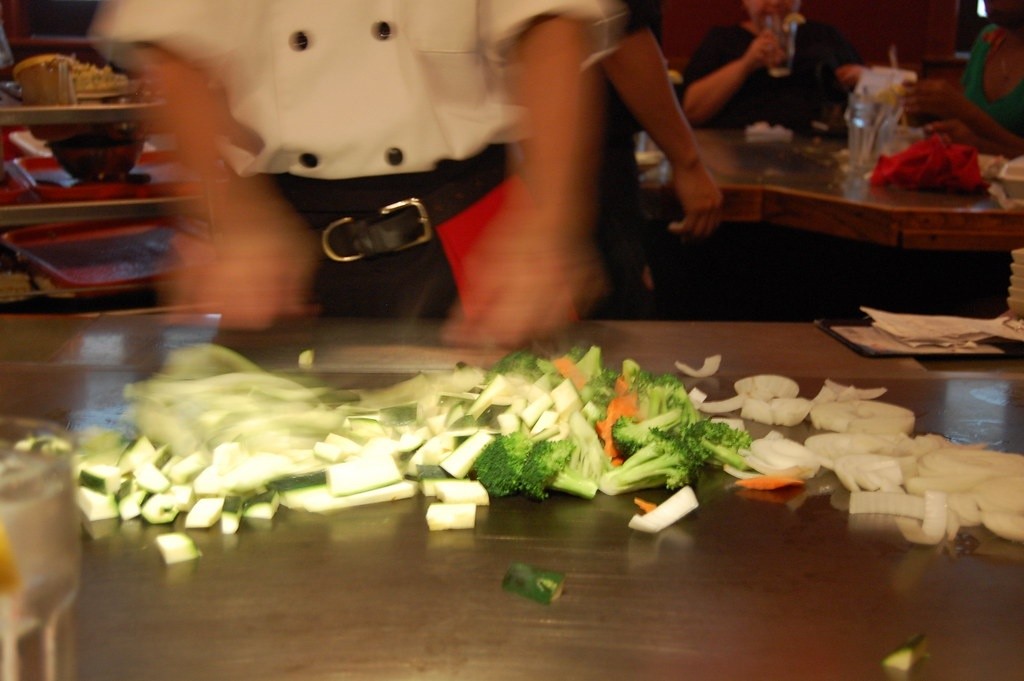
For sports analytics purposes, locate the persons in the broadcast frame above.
[583,0,724,322]
[897,0,1024,160]
[90,0,619,351]
[683,0,866,139]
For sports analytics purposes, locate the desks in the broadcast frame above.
[634,127,1024,252]
[0,314,1024,680]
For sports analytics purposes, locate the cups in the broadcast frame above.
[635,130,667,184]
[848,83,906,169]
[760,12,797,75]
[0,417,79,681]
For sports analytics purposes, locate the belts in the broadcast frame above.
[311,164,506,264]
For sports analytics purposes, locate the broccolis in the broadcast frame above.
[467,345,754,498]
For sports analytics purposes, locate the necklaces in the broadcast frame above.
[999,53,1011,81]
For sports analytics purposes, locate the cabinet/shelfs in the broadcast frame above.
[1,106,212,306]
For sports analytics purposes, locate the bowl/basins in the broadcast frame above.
[994,177,1024,198]
[0,312,222,408]
[44,134,145,184]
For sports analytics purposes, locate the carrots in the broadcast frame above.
[549,356,806,515]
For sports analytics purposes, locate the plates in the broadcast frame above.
[0,81,165,126]
[0,198,198,223]
[1006,247,1024,316]
